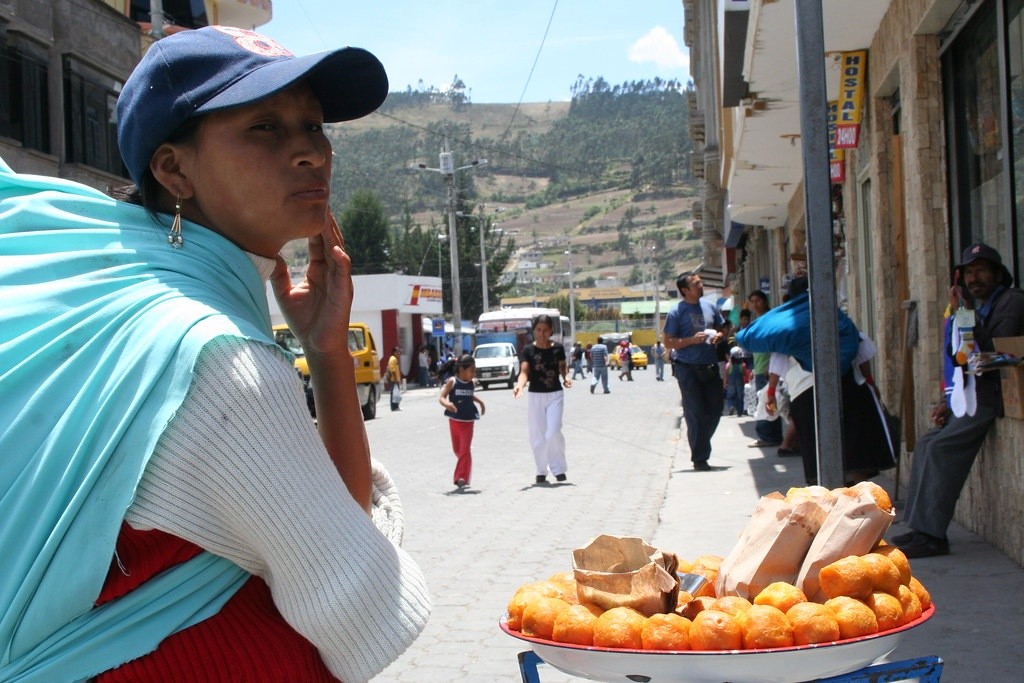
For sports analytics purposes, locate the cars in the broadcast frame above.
[609,343,648,370]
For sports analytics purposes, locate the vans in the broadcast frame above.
[273,322,380,419]
[473,343,519,390]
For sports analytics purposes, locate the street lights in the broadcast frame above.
[456,207,505,313]
[629,242,646,329]
[564,251,576,346]
[410,159,488,357]
[437,234,447,362]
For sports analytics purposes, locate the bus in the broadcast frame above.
[475,306,571,373]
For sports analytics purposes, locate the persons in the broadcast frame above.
[77,24,432,683]
[388,347,400,411]
[570,342,592,380]
[590,337,610,394]
[718,277,898,488]
[663,272,729,472]
[418,344,455,388]
[651,340,666,381]
[514,314,573,483]
[436,355,485,489]
[891,244,1024,560]
[618,340,634,382]
[668,348,676,376]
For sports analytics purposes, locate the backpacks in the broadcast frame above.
[384,368,391,382]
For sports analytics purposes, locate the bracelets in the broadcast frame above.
[767,388,775,396]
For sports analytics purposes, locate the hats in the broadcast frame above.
[729,346,744,359]
[954,243,1002,271]
[620,341,627,346]
[116,25,391,190]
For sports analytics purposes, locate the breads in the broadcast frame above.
[506,542,929,651]
[785,481,892,516]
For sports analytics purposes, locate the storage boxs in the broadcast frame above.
[968,337,1024,419]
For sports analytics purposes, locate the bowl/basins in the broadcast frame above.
[499,603,936,683]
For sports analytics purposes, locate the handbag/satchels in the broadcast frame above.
[392,384,402,403]
[674,360,719,382]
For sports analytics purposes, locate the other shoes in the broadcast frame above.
[458,478,466,489]
[556,474,566,481]
[536,474,546,483]
[604,390,610,393]
[729,406,735,415]
[777,447,803,456]
[891,530,919,545]
[899,533,951,559]
[748,438,778,447]
[591,385,594,393]
[694,460,711,471]
[737,411,742,417]
